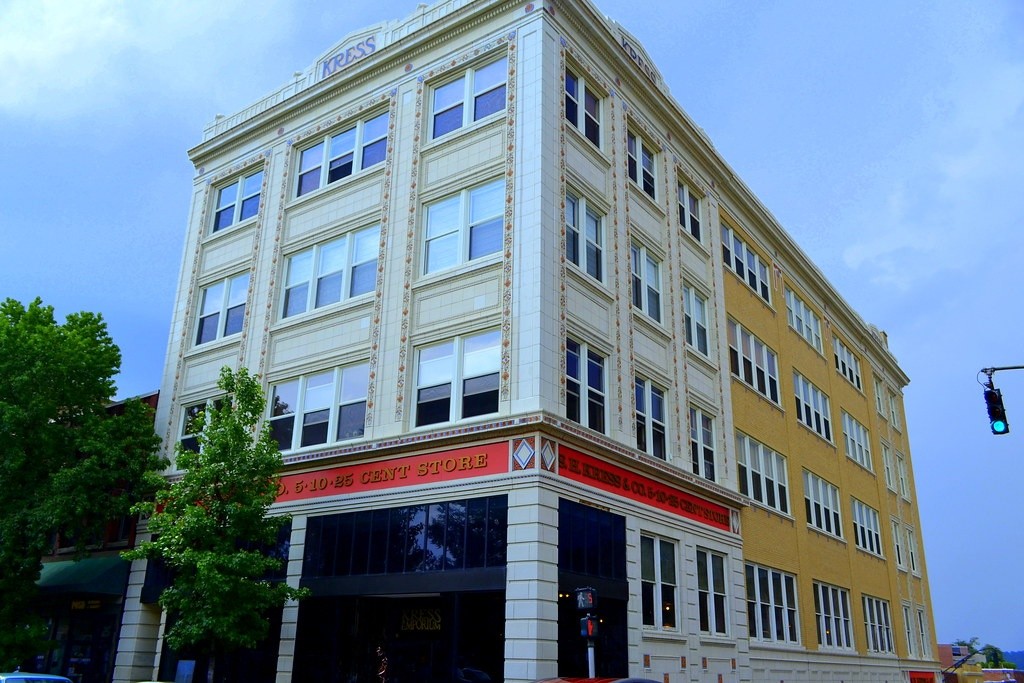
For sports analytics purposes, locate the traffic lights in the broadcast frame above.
[582,616,599,637]
[992,653,999,667]
[984,388,1010,434]
[576,587,598,609]
